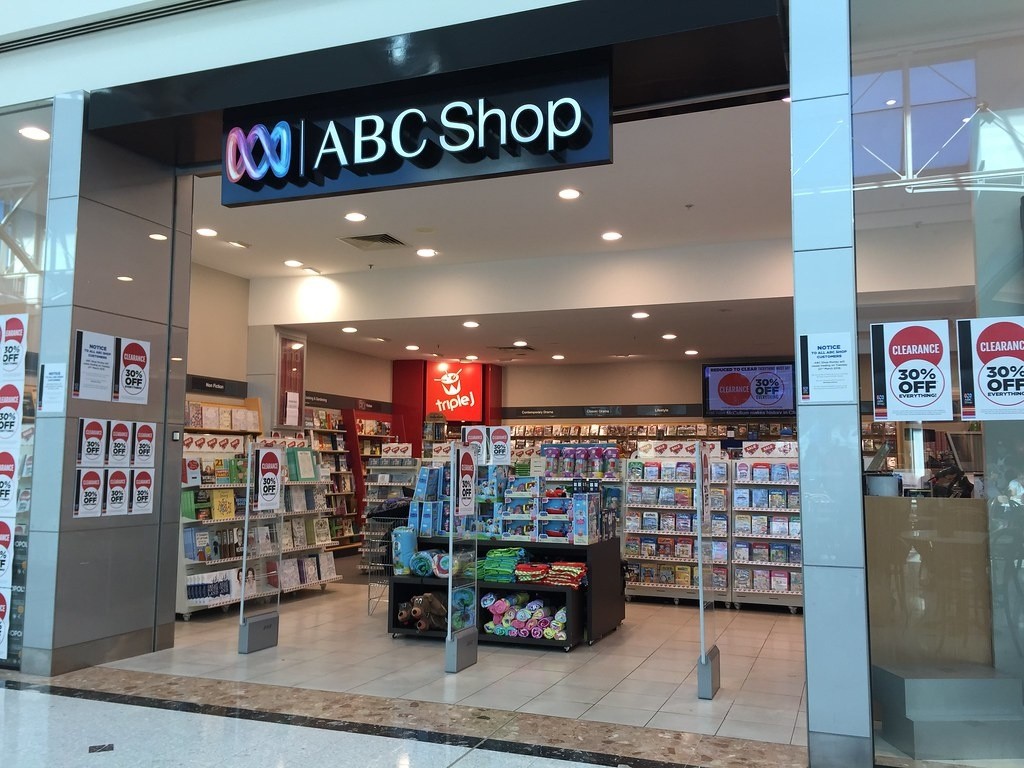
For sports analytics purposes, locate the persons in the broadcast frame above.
[246,567,256,594]
[237,568,242,595]
[234,528,244,556]
[984,420,1024,501]
[937,453,945,468]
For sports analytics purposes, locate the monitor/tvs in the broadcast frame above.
[945,431,984,475]
[701,363,797,419]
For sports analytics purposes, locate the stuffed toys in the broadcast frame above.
[397,592,448,632]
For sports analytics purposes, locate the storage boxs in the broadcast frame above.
[359,446,803,591]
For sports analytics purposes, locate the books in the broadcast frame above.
[181,446,338,608]
[356,418,391,476]
[305,407,360,546]
[184,400,260,433]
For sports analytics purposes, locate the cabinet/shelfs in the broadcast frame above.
[387,535,626,654]
[731,457,802,615]
[342,409,406,546]
[355,455,421,575]
[624,452,731,609]
[175,433,343,622]
[305,427,363,552]
